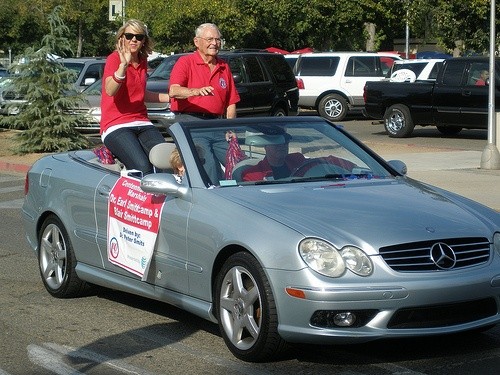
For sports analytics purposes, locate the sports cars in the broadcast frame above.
[21,116,499,363]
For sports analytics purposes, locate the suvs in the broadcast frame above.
[381,58,445,85]
[148,48,299,119]
[292,51,405,122]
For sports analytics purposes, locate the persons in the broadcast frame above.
[99,18,170,180]
[171,144,210,186]
[241,126,360,182]
[168,22,245,185]
[476,70,489,86]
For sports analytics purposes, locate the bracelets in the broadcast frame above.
[113,71,126,83]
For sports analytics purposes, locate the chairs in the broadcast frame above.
[148,142,177,173]
[231,158,262,182]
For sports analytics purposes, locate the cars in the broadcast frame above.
[0,57,168,135]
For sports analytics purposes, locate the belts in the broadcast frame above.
[174,111,221,120]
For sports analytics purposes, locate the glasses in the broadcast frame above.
[123,33,145,41]
[198,37,222,43]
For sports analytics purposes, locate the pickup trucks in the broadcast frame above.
[363,55,498,138]
